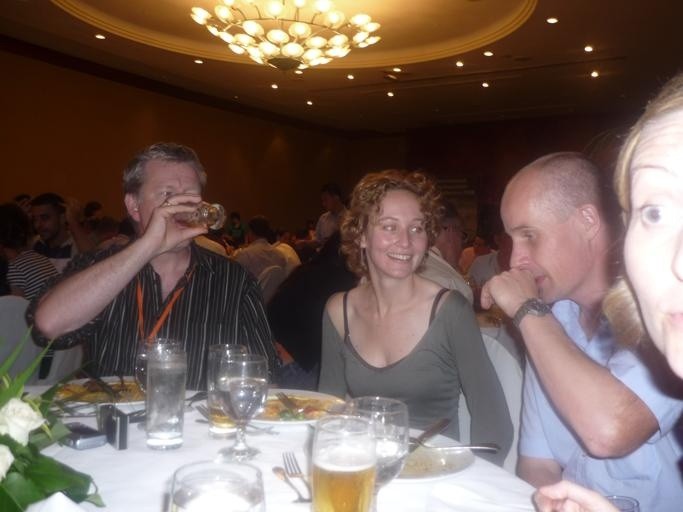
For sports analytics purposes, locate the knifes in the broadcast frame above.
[401,417,451,461]
[79,366,121,398]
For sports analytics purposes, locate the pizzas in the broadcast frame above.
[252,395,334,421]
[49,384,148,402]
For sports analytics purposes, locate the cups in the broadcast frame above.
[602,494,639,510]
[173,197,226,232]
[310,416,374,512]
[144,351,185,450]
[165,460,268,511]
[206,344,249,437]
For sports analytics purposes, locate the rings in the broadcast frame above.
[164,200,173,206]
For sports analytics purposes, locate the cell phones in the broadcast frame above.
[55,422,108,450]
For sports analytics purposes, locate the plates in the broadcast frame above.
[249,387,346,425]
[59,380,144,405]
[392,429,477,481]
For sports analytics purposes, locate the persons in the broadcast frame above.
[25,140,287,395]
[531,71,683,512]
[0,183,513,368]
[478,147,679,512]
[317,166,515,470]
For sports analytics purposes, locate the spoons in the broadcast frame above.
[272,467,310,505]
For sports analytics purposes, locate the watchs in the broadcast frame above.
[513,296,553,328]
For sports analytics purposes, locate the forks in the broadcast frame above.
[194,403,276,434]
[273,391,341,416]
[282,450,310,493]
[113,368,131,393]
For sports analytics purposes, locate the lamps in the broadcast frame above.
[191,0,382,74]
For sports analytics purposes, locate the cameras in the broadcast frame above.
[100,404,128,450]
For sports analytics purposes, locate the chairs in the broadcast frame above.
[1,262,553,511]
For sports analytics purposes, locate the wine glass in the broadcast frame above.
[215,353,269,458]
[135,338,182,432]
[344,394,408,512]
